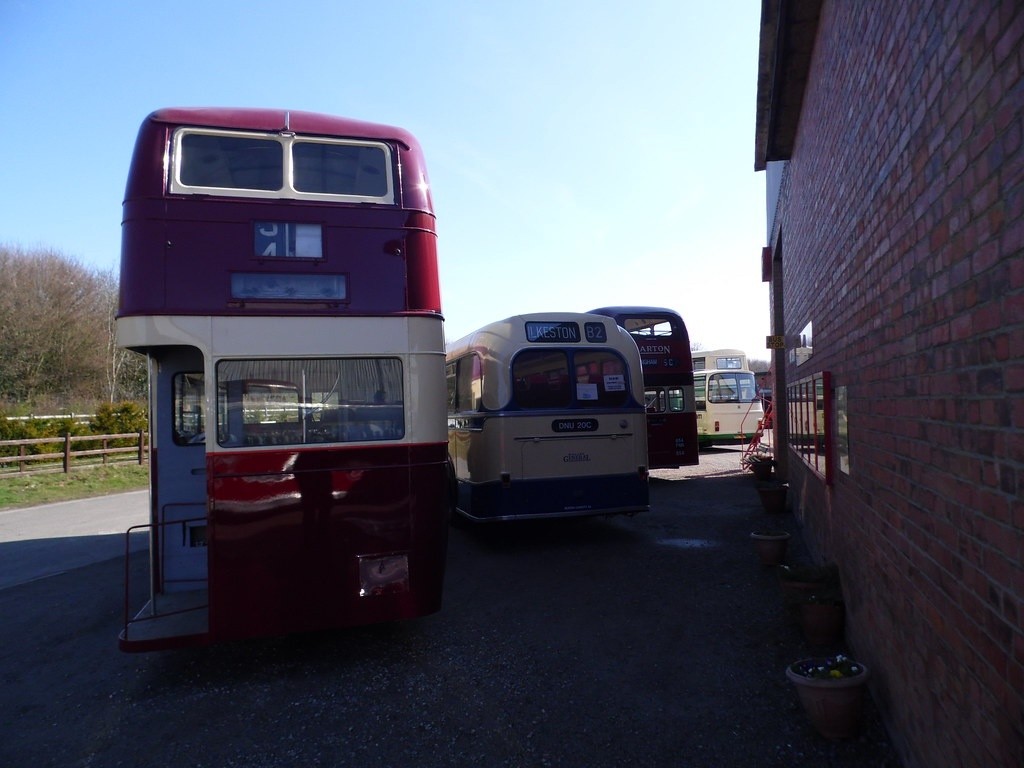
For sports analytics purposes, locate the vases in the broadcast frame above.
[784,658,871,742]
[758,488,787,513]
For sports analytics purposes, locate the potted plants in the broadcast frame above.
[783,565,836,598]
[750,529,793,566]
[752,457,778,478]
[800,596,844,646]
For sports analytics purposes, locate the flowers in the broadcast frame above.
[795,655,861,679]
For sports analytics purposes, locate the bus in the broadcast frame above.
[590,304,702,469]
[446,312,653,526]
[110,103,453,654]
[692,350,749,372]
[694,369,765,449]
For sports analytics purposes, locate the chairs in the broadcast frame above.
[518,373,619,405]
